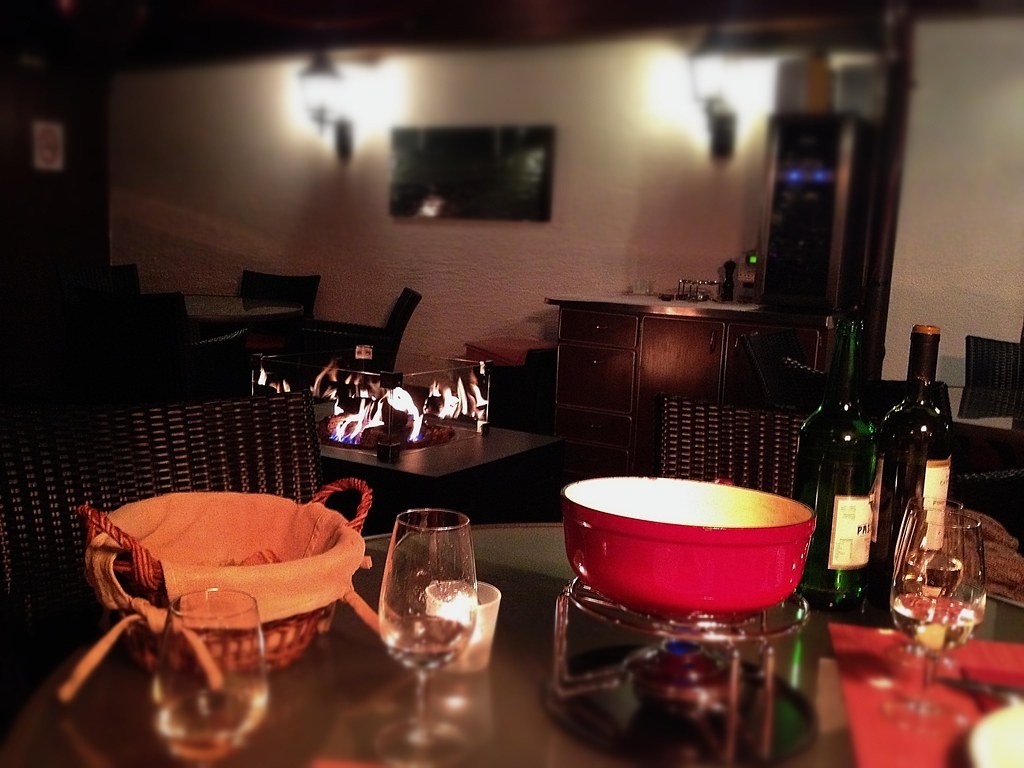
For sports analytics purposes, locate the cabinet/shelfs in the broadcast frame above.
[544,294,842,490]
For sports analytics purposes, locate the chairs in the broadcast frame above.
[963,333,1024,383]
[956,385,1024,420]
[56,262,422,409]
[0,388,319,744]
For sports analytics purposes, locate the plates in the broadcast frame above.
[965,703,1024,768]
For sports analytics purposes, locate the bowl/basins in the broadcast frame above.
[559,475,818,624]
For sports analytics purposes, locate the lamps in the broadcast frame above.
[689,24,737,162]
[300,49,354,164]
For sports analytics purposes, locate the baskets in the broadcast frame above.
[78,477,374,673]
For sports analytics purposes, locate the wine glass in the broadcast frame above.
[371,509,479,768]
[882,498,980,735]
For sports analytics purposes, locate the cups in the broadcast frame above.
[147,587,271,768]
[425,581,503,674]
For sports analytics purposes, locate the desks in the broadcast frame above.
[941,384,1024,439]
[320,425,565,486]
[183,291,305,337]
[0,524,1022,765]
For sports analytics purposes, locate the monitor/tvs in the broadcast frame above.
[389,125,553,220]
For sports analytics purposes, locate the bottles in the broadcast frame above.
[790,317,879,616]
[851,324,953,626]
